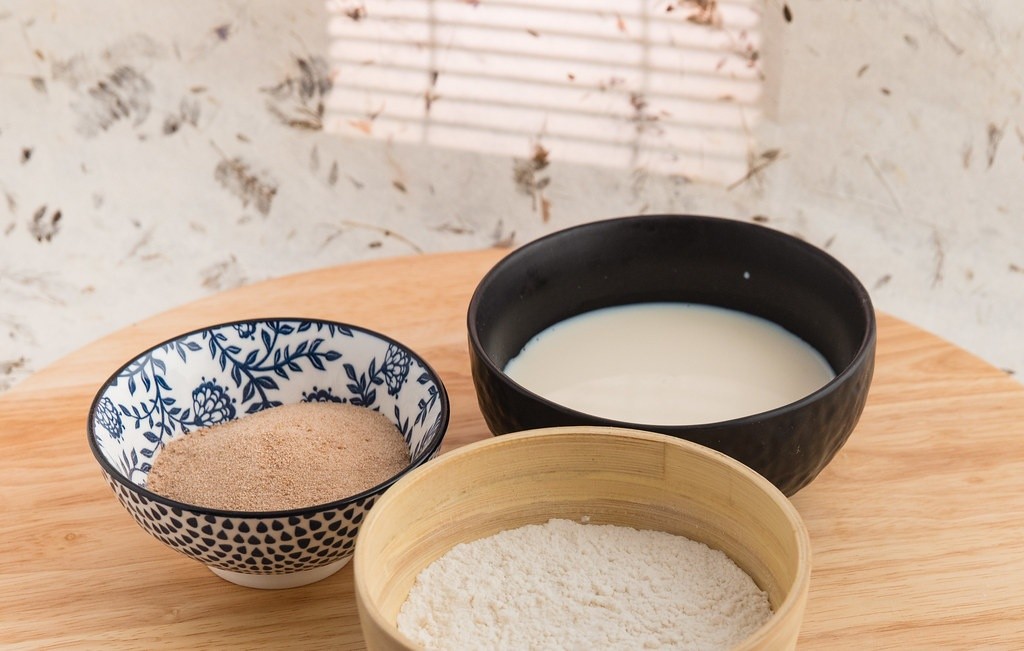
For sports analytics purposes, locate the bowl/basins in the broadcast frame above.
[353,426,813,651]
[85,317,450,589]
[466,214,876,498]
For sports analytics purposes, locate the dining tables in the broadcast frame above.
[0,244,1024,651]
